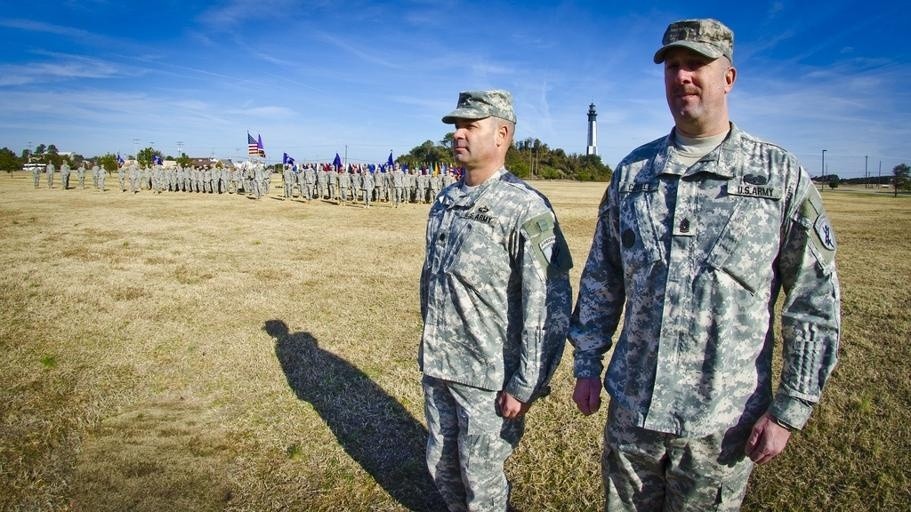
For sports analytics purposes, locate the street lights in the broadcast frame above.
[821,148,828,191]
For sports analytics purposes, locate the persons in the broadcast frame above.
[247,134,258,157]
[46,160,55,189]
[31,163,41,188]
[61,159,71,189]
[566,19,843,512]
[77,163,86,189]
[256,134,265,158]
[282,151,294,166]
[92,162,100,187]
[418,92,574,512]
[152,155,163,165]
[116,155,124,166]
[99,164,108,192]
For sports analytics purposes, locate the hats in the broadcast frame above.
[442,88,518,126]
[262,321,282,328]
[653,17,737,67]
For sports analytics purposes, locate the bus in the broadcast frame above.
[22,163,46,172]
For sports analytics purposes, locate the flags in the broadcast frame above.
[332,152,342,173]
[386,151,394,165]
